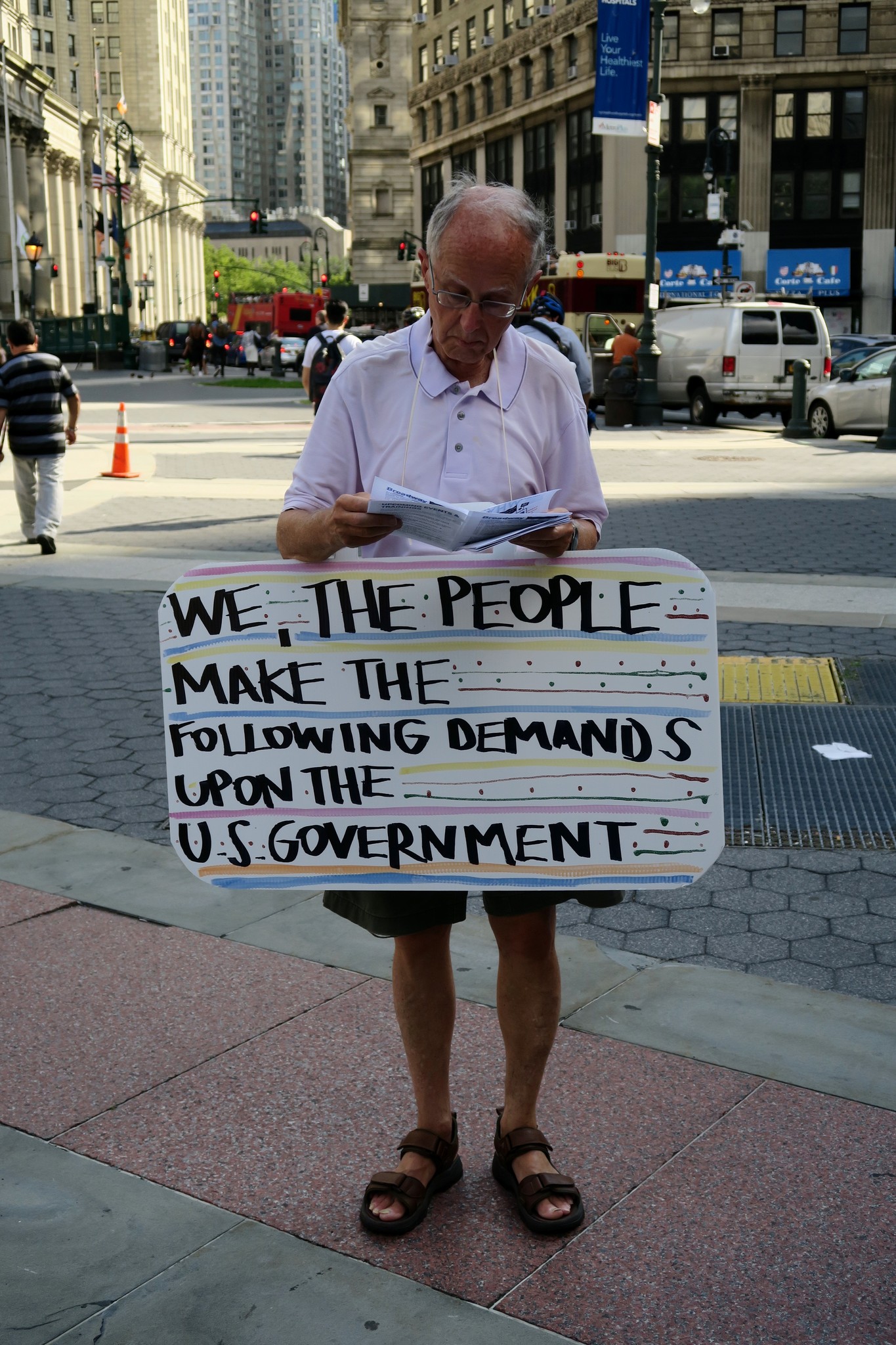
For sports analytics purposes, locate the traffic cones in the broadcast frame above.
[100,402,139,480]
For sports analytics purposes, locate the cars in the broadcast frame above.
[806,344,896,439]
[154,319,390,372]
[830,347,887,383]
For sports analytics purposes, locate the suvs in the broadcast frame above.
[830,332,896,362]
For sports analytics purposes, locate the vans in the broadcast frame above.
[5,313,125,370]
[583,302,831,430]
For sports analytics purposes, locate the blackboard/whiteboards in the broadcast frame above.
[159,548,730,892]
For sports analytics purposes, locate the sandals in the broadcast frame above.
[490,1106,587,1241]
[362,1115,465,1241]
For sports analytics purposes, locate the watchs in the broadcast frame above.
[567,522,578,551]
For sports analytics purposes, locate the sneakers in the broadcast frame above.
[25,537,39,544]
[38,534,57,555]
[190,367,195,375]
[247,372,251,376]
[179,365,185,372]
[214,368,221,377]
[199,371,203,377]
[251,372,255,375]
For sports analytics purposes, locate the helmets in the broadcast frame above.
[529,292,565,325]
[403,306,426,320]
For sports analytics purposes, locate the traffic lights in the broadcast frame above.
[397,239,406,260]
[249,210,258,235]
[282,287,288,293]
[259,213,269,234]
[50,264,59,278]
[321,273,327,288]
[214,292,220,300]
[214,270,219,282]
[406,241,417,261]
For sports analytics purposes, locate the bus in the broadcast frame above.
[411,250,662,336]
[228,290,325,340]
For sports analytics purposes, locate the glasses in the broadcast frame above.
[426,257,530,317]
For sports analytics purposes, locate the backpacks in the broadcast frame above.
[310,331,355,411]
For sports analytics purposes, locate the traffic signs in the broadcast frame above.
[712,276,739,286]
[135,280,156,287]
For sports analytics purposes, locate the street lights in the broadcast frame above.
[703,125,736,302]
[24,230,44,330]
[298,240,313,294]
[100,119,142,370]
[78,200,99,313]
[310,226,331,303]
[633,0,710,420]
[103,254,116,368]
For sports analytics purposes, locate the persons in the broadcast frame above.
[276,173,626,1241]
[210,314,227,377]
[0,318,80,556]
[180,336,195,375]
[305,310,330,346]
[189,317,204,377]
[611,321,640,370]
[516,292,592,410]
[201,340,208,374]
[241,323,262,376]
[401,307,426,328]
[300,298,362,415]
